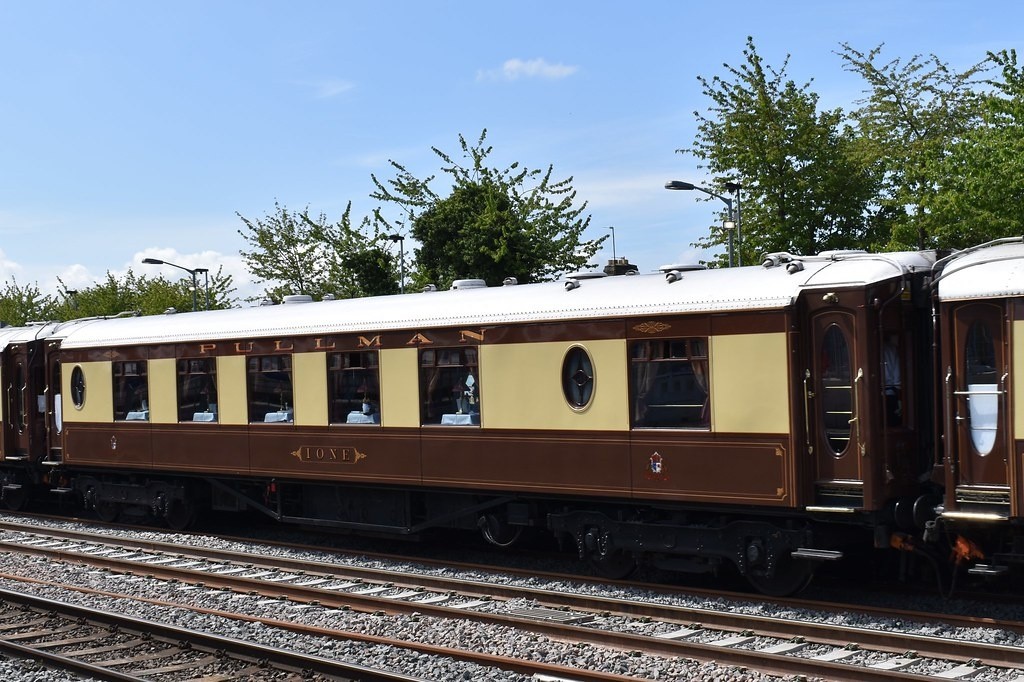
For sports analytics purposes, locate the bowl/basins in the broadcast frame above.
[456,411,462,415]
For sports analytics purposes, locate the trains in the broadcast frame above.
[0,236,1024,616]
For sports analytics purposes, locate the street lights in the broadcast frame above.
[663,180,742,268]
[380,233,406,294]
[141,258,208,312]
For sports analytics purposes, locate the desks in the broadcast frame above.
[441,414,480,425]
[193,413,218,421]
[264,411,293,422]
[346,411,380,424]
[125,408,149,421]
